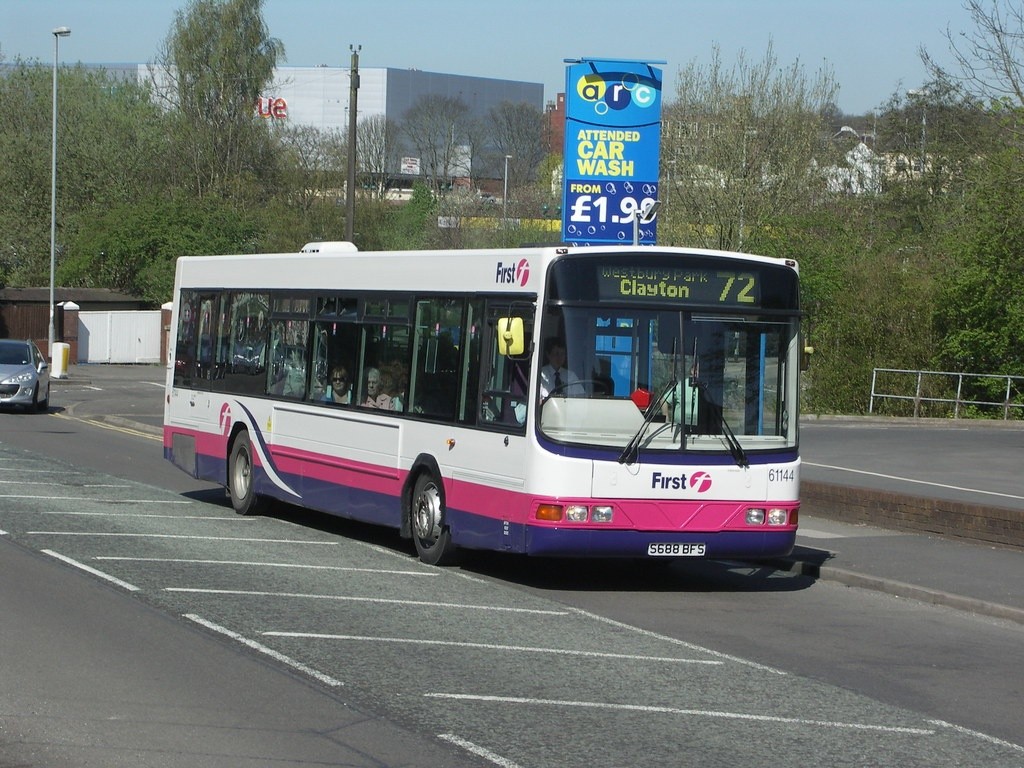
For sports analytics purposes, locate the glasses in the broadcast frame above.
[332,376,345,382]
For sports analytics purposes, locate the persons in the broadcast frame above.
[281,332,460,416]
[537,338,586,408]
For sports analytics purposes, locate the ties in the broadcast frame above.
[554,372,563,395]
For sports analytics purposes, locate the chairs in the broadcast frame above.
[174,335,477,417]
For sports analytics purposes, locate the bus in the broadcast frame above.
[163,240,812,569]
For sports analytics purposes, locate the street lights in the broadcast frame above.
[738,128,759,253]
[504,154,512,218]
[666,161,678,234]
[47,27,72,363]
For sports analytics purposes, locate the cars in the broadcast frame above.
[0,336,50,412]
